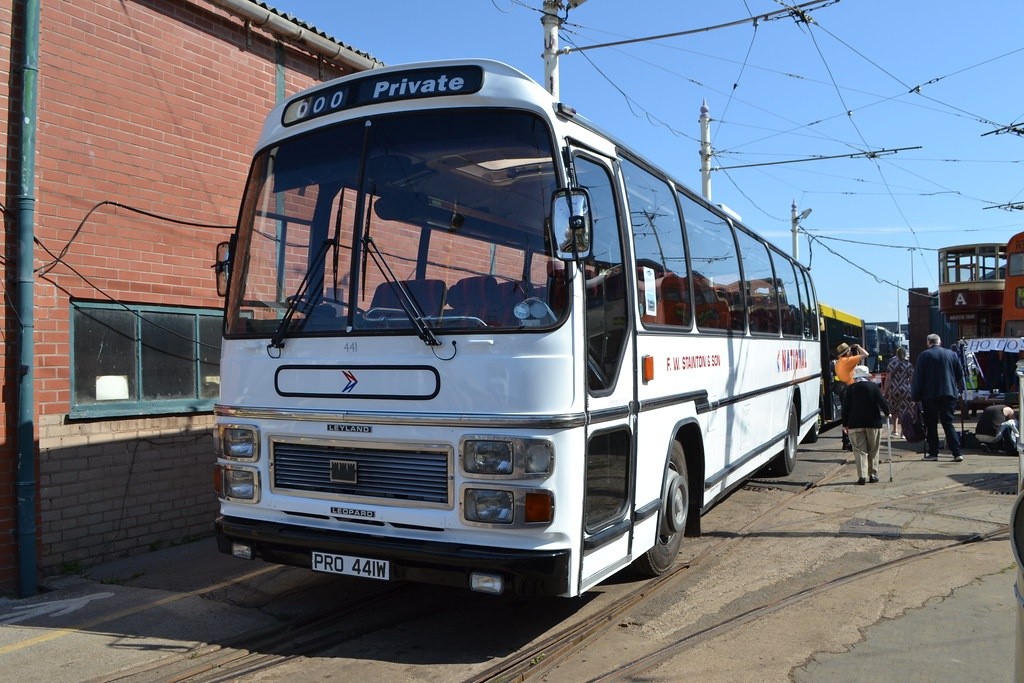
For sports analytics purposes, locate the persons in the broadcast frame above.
[911,334,963,462]
[882,346,915,439]
[834,343,870,452]
[950,339,963,359]
[841,364,893,485]
[974,402,1017,454]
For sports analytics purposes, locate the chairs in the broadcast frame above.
[718,285,798,334]
[365,276,548,328]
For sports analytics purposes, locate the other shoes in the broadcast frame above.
[869,476,879,483]
[921,454,937,461]
[891,431,896,435]
[954,456,963,461]
[980,443,992,452]
[858,477,865,485]
[843,442,852,450]
[998,449,1008,454]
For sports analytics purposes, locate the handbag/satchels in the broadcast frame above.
[832,381,847,397]
[900,402,927,443]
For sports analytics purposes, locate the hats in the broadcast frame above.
[853,365,872,379]
[837,343,851,357]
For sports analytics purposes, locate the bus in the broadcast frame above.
[216,56,822,608]
[998,230,1024,393]
[865,325,896,373]
[816,302,865,427]
